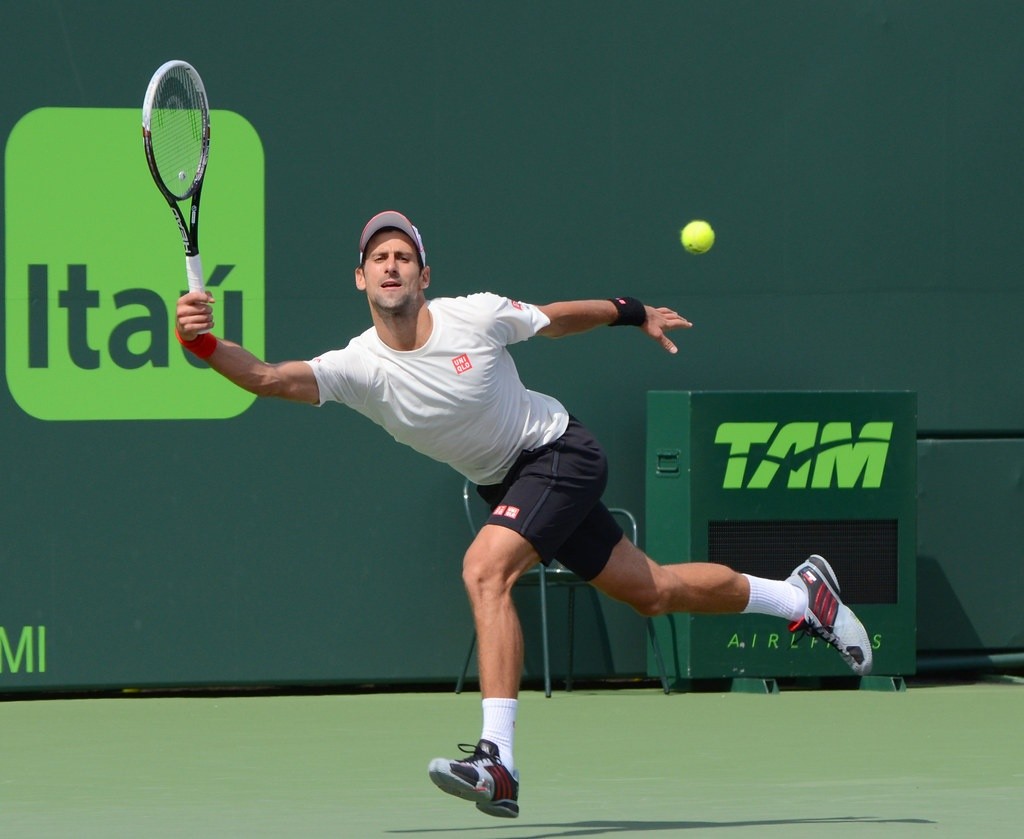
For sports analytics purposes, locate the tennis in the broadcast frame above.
[680,220,715,255]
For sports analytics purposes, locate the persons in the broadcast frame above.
[173,210,873,818]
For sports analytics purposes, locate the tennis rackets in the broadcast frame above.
[140,56,217,335]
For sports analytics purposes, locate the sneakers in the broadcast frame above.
[427,738,520,819]
[784,554,873,675]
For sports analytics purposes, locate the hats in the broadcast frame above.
[357,210,426,268]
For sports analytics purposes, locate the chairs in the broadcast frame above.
[454,479,673,700]
